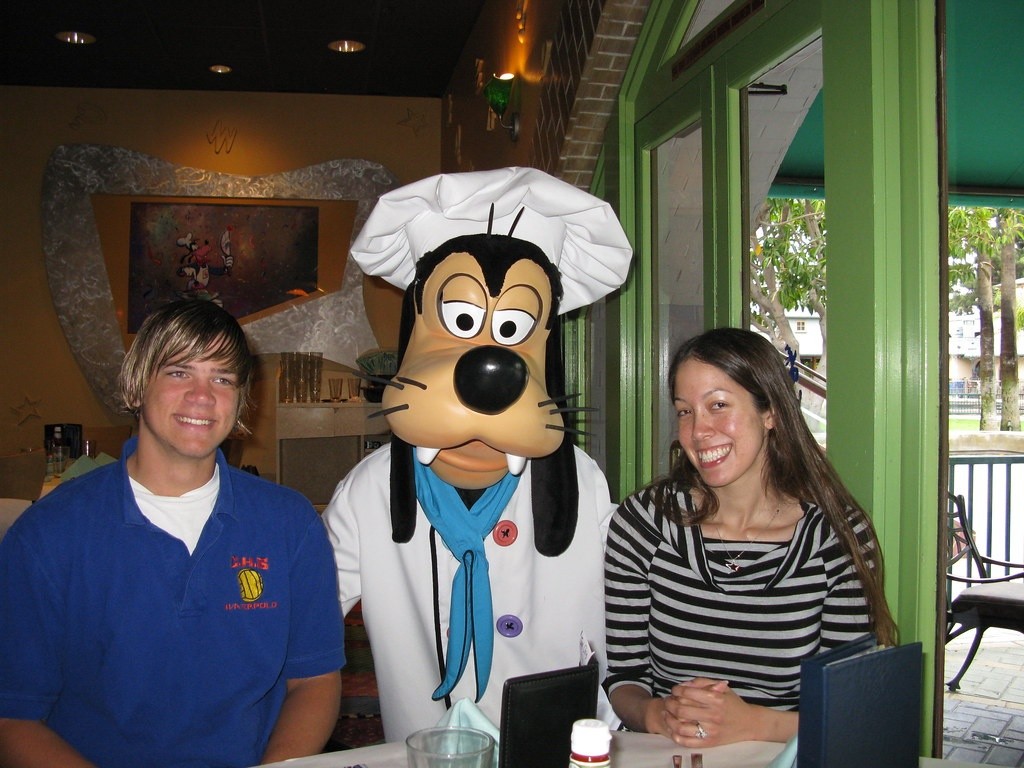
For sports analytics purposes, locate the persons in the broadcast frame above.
[0,297,347,768]
[600,327,898,750]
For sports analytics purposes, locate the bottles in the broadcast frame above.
[569,719,612,767]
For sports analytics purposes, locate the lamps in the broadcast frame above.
[483,72,520,142]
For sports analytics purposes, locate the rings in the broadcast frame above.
[696,724,707,739]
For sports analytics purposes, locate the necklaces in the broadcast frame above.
[710,505,780,574]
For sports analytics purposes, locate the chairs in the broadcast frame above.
[945,492,1024,694]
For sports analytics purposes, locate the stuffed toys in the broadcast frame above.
[319,167,629,746]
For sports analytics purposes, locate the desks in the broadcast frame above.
[278,402,390,511]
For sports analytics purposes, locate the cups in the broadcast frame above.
[279,351,323,403]
[348,378,361,401]
[329,378,343,400]
[52,447,70,477]
[82,440,95,459]
[406,726,494,768]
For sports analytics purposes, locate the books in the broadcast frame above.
[797,633,922,768]
